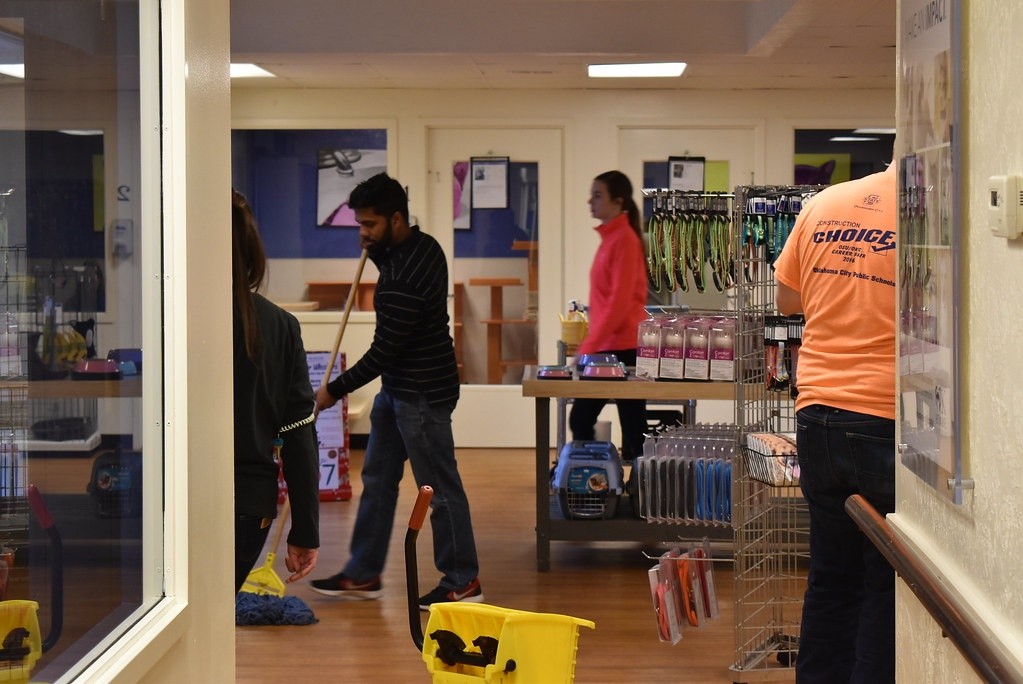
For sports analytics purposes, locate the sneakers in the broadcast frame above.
[418,578,483,611]
[309,572,381,600]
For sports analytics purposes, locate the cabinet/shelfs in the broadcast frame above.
[522,363,811,570]
[0,378,141,566]
[730,187,827,684]
[289,310,386,434]
[0,247,103,531]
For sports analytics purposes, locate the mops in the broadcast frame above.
[201,195,374,627]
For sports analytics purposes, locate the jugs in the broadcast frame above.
[558,311,590,357]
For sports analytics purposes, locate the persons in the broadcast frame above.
[309,170,485,611]
[231,188,321,598]
[771,137,899,683]
[568,170,650,465]
